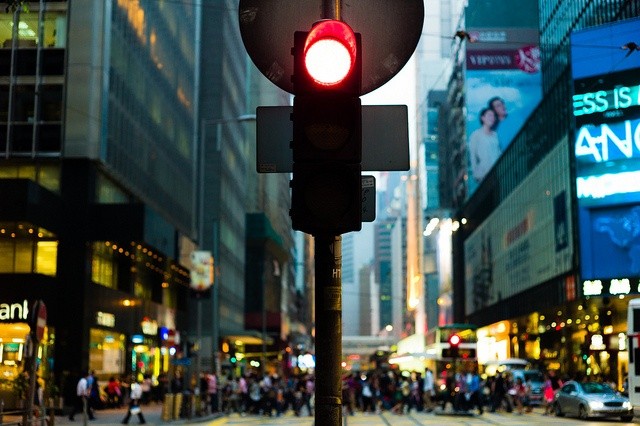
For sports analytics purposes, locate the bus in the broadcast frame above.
[627,297,640,406]
[397,323,478,379]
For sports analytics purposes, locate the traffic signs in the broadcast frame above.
[238,0,424,96]
[256,104,410,174]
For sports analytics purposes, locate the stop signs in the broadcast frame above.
[31,300,47,342]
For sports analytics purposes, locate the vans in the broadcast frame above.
[512,370,544,407]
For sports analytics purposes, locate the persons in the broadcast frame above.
[541,369,563,416]
[142,370,154,401]
[469,107,503,185]
[342,365,534,416]
[489,97,520,151]
[68,371,96,421]
[122,380,146,424]
[107,376,122,408]
[22,369,32,426]
[86,369,95,395]
[33,374,47,426]
[197,368,317,417]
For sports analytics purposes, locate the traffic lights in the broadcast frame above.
[447,335,460,360]
[289,19,361,238]
[226,345,237,365]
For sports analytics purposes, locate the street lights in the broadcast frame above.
[197,114,256,373]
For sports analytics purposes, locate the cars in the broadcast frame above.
[553,381,634,422]
